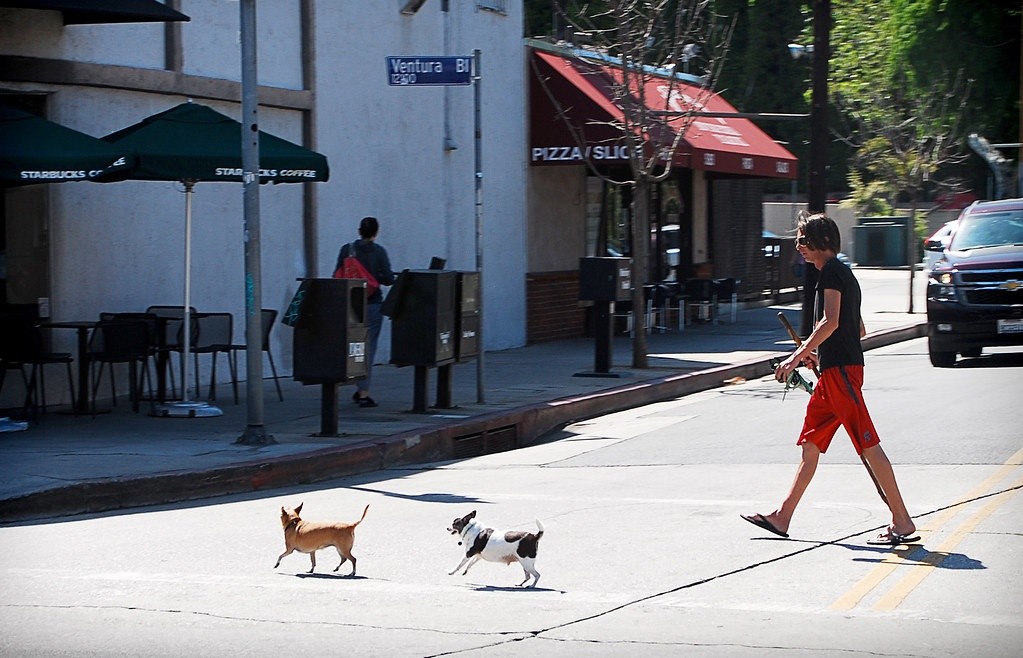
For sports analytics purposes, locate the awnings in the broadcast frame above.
[529,49,797,176]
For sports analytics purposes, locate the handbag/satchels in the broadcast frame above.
[331,243,379,299]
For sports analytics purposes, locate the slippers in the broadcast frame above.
[739,513,790,538]
[866,534,921,545]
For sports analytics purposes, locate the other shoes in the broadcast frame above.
[352,390,380,408]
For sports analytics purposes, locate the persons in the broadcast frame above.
[738,212,922,545]
[332,217,395,409]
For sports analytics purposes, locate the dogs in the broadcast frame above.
[273,502,370,577]
[447,510,545,589]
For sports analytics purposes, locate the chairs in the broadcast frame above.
[0,303,285,415]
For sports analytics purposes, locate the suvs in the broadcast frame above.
[923,198,1023,368]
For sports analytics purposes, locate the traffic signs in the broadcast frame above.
[385,53,471,87]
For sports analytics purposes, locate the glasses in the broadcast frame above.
[794,235,807,248]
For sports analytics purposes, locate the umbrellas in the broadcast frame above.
[87,94,331,420]
[1,108,130,439]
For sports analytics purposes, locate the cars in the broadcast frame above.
[758,231,850,271]
[652,224,690,268]
[923,218,960,270]
